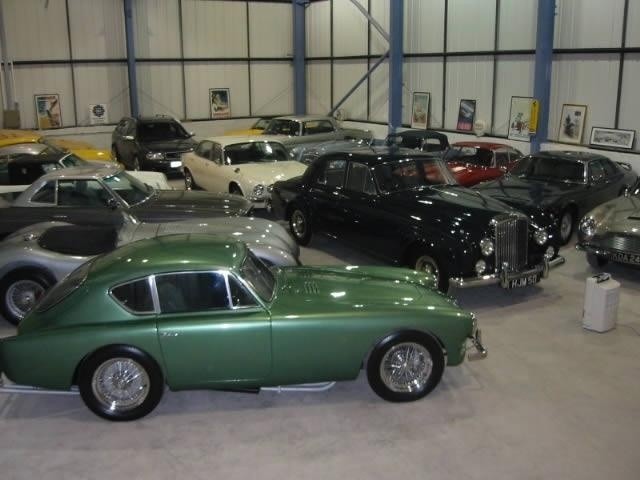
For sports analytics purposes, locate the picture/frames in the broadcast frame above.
[411,91,430,131]
[589,126,637,150]
[507,95,533,142]
[34,94,63,130]
[557,104,587,145]
[457,99,476,132]
[209,87,231,119]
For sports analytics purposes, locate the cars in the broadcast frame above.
[180,137,309,211]
[253,115,375,151]
[219,114,320,146]
[287,150,567,300]
[0,143,256,233]
[469,149,640,247]
[384,130,451,156]
[1,232,485,422]
[0,130,117,162]
[402,140,526,191]
[0,214,302,328]
[573,177,640,266]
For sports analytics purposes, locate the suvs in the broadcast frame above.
[112,114,201,179]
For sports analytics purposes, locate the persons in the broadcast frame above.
[511,110,525,134]
